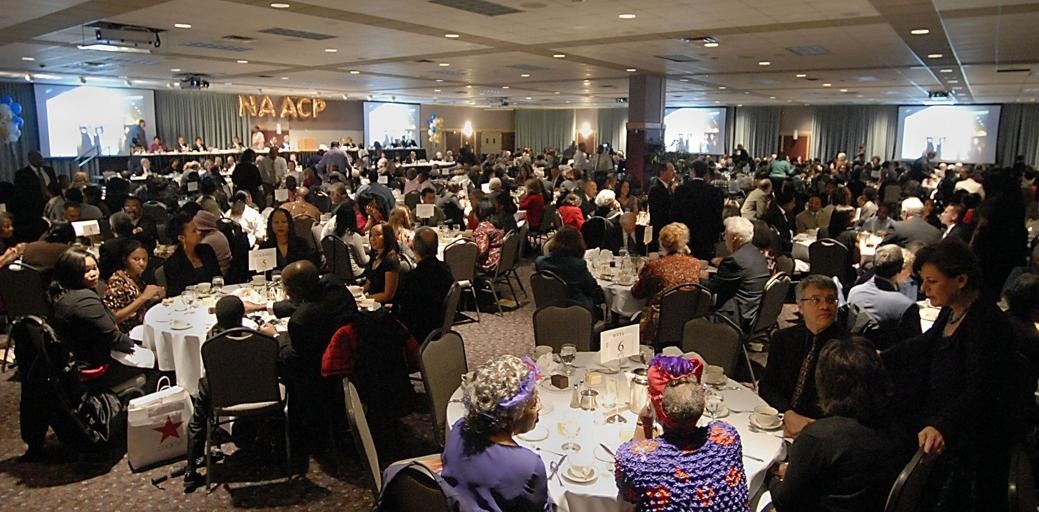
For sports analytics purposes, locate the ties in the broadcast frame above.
[628,233,634,253]
[38,168,45,199]
[791,337,816,408]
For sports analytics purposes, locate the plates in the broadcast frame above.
[749,414,783,429]
[169,324,193,330]
[542,379,571,391]
[517,424,549,440]
[250,281,265,285]
[562,465,599,483]
[594,443,616,462]
[705,376,727,384]
[353,293,362,297]
[703,408,729,417]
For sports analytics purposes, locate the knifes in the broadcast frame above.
[548,455,567,480]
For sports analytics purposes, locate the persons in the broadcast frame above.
[1,120,1039,512]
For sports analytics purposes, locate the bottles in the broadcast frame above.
[570,379,585,408]
[630,367,650,414]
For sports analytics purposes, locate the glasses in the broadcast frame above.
[800,296,838,304]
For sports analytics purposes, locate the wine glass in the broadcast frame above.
[182,285,199,314]
[705,392,725,421]
[557,414,582,453]
[638,346,654,368]
[271,270,281,282]
[212,276,224,299]
[560,344,576,376]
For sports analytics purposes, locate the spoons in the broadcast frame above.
[549,461,563,487]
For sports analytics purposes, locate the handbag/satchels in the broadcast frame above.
[127,376,195,470]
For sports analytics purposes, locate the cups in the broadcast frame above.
[348,285,364,294]
[754,407,785,425]
[252,275,265,283]
[567,454,594,478]
[197,282,212,293]
[706,365,724,380]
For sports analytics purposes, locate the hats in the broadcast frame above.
[193,210,217,231]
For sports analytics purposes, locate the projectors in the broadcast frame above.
[72,25,154,55]
[928,90,949,102]
[178,77,209,89]
[492,98,511,108]
[615,96,627,104]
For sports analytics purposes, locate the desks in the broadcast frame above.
[44,149,427,189]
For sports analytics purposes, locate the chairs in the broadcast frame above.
[151,263,166,298]
[747,272,791,356]
[515,220,530,298]
[418,328,470,451]
[656,282,713,348]
[881,446,929,511]
[464,228,520,316]
[1006,453,1039,512]
[681,312,744,376]
[808,237,847,274]
[342,378,445,503]
[532,301,592,354]
[554,211,563,229]
[840,299,877,339]
[200,326,287,492]
[14,323,146,446]
[440,282,461,333]
[529,270,606,336]
[443,237,481,322]
[322,235,363,284]
[541,238,557,257]
[384,465,453,512]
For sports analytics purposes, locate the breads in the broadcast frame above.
[551,374,568,389]
[201,298,212,306]
[707,364,724,383]
[585,371,601,386]
[161,298,174,307]
